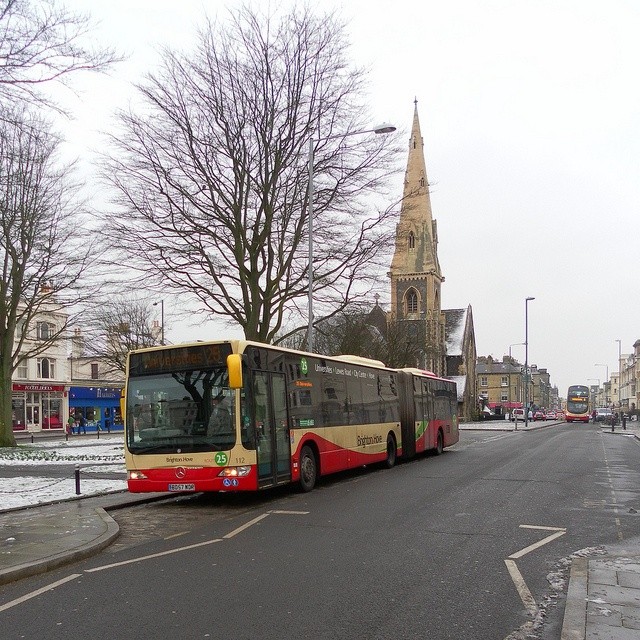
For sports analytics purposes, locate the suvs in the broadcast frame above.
[511,408,525,422]
[595,408,612,422]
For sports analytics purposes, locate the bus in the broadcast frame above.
[122,340,460,493]
[565,385,590,422]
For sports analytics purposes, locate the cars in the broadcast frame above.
[546,411,559,421]
[533,411,546,421]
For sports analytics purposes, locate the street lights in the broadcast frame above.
[525,296,536,427]
[152,300,165,345]
[508,342,528,420]
[614,339,621,417]
[305,123,397,353]
[587,378,601,406]
[593,362,609,415]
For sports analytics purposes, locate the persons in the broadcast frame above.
[78,413,85,434]
[68,416,75,431]
